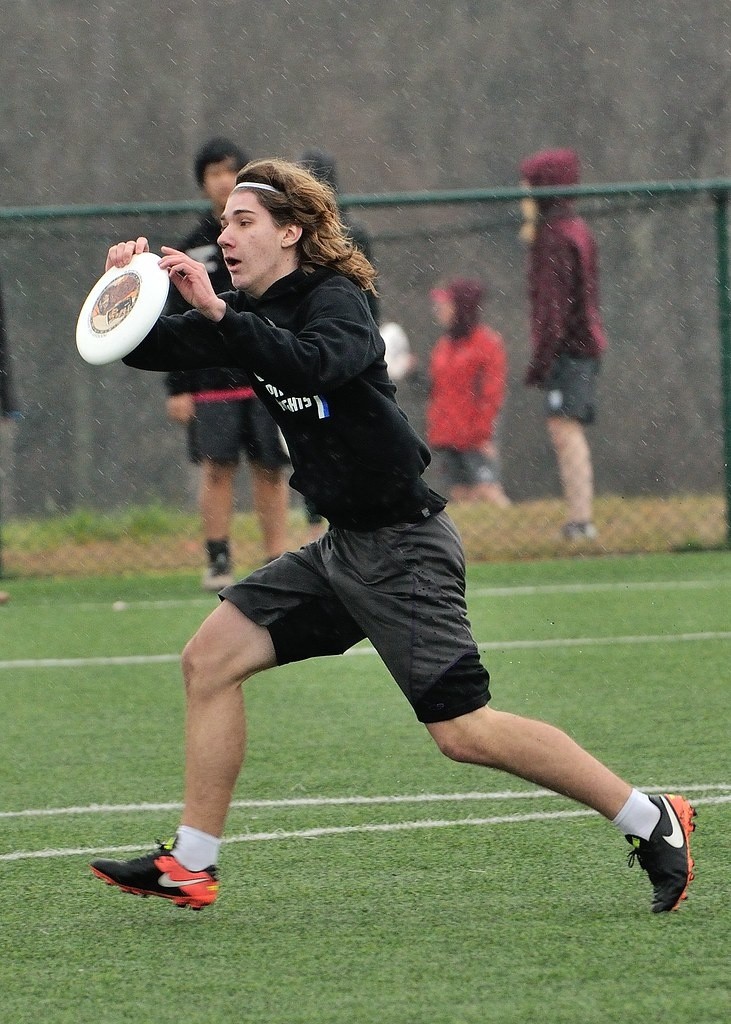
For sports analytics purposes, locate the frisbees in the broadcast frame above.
[379,320,411,381]
[75,251,171,367]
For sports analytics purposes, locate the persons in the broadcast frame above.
[523,150,609,541]
[89,160,697,914]
[299,146,373,263]
[400,279,512,508]
[164,137,292,589]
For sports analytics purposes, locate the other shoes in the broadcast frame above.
[205,551,236,590]
[549,521,597,543]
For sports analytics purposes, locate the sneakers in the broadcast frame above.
[625,793,697,914]
[88,833,220,912]
